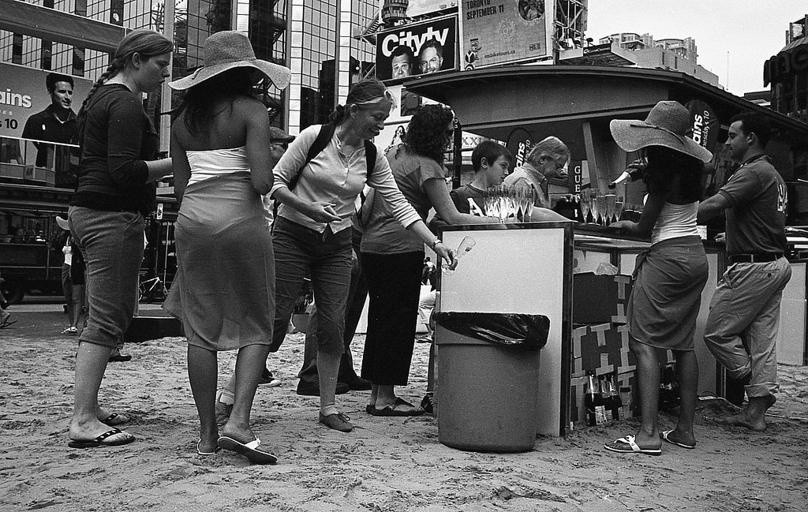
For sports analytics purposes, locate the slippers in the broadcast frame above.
[604,435,662,455]
[660,429,694,449]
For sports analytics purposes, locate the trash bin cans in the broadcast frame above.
[433,310,551,454]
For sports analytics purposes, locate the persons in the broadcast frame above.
[501,135,569,223]
[417,39,444,74]
[696,111,793,432]
[67,28,173,447]
[421,257,436,286]
[387,125,406,153]
[257,125,298,391]
[161,30,292,465]
[359,102,522,417]
[54,214,148,362]
[22,73,78,145]
[604,98,714,455]
[420,140,601,415]
[389,44,414,79]
[216,75,458,432]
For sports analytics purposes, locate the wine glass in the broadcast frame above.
[580,188,623,227]
[441,236,476,275]
[484,184,535,224]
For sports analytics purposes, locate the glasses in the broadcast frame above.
[358,90,394,104]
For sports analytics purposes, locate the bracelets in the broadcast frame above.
[432,239,442,250]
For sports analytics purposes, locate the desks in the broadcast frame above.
[435,221,745,438]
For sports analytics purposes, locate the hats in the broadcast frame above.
[168,31,291,90]
[610,100,713,164]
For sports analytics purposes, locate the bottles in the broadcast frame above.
[584,361,678,426]
[608,163,644,189]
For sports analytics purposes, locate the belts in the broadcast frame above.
[728,252,785,263]
